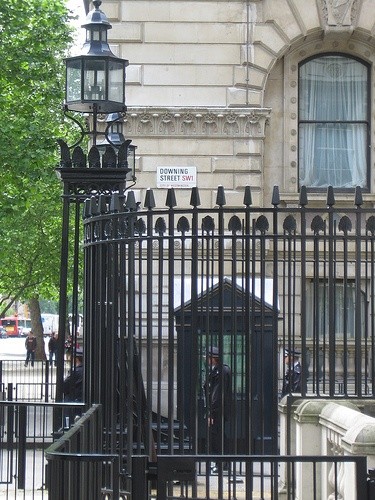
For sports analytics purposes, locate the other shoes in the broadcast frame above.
[24,363,27,367]
[213,467,219,472]
[211,465,217,469]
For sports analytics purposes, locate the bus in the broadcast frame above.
[0,316,31,336]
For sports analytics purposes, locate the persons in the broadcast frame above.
[64,349,86,403]
[48,330,60,367]
[200,347,234,474]
[281,348,309,405]
[24,330,36,368]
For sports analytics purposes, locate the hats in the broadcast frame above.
[205,346,220,358]
[284,348,300,357]
[73,349,83,356]
